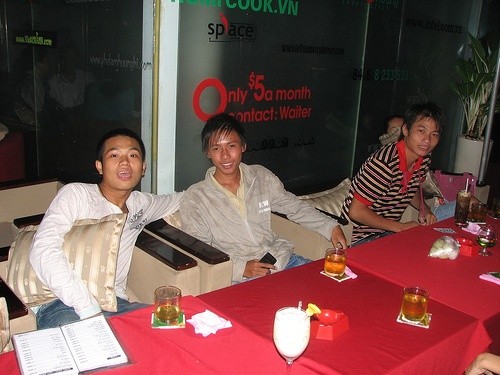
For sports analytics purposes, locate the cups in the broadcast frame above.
[454,189,473,227]
[324,247,347,279]
[401,285,430,324]
[472,202,488,222]
[154,285,182,325]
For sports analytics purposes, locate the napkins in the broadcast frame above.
[185,307,234,337]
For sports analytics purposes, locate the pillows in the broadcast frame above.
[5,211,127,314]
[296,176,351,218]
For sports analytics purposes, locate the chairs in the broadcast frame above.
[147,209,353,295]
[0,173,201,351]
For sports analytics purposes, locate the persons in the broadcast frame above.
[179,112,348,286]
[28,128,185,330]
[384,114,456,227]
[341,104,442,248]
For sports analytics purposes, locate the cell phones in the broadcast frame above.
[260,252,276,270]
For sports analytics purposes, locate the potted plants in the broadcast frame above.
[449,32,500,182]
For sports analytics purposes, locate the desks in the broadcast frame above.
[1,206,500,375]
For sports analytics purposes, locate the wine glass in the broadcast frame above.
[477,225,497,257]
[273,307,311,375]
